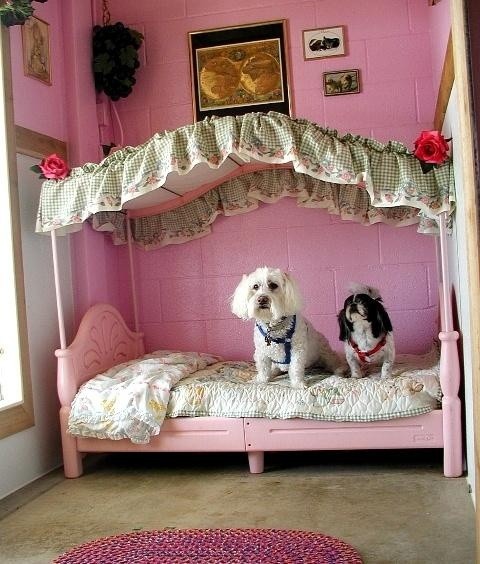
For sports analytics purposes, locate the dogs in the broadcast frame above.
[227,264,351,390]
[336,293,396,380]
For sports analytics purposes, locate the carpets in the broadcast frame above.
[52,528,364,564]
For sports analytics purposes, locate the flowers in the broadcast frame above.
[412,129,454,175]
[28,153,70,181]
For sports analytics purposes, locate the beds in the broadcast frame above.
[36,110,465,478]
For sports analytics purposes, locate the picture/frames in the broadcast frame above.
[21,15,52,86]
[187,17,293,125]
[323,68,360,96]
[301,25,346,61]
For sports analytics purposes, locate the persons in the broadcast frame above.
[29,23,48,77]
